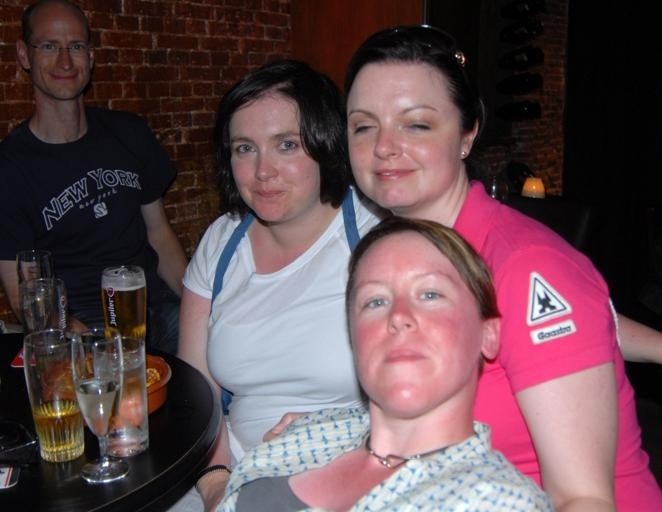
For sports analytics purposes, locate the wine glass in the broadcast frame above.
[71,327,131,484]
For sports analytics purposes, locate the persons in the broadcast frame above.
[210,215,555,512]
[163,60,661,511]
[342,24,662,510]
[0,2,192,357]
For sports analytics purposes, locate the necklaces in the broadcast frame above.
[363,434,456,469]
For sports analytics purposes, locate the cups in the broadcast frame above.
[92,335,149,458]
[15,250,86,462]
[101,264,147,353]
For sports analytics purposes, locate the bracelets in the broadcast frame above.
[194,465,232,486]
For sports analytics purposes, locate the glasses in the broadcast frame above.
[373,23,465,64]
[31,40,92,57]
